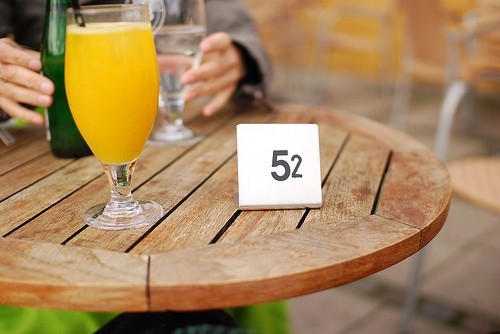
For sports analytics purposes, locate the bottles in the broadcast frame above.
[40,0,94,158]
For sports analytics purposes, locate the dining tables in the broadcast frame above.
[0,98,453,312]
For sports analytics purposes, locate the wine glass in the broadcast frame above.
[133,0,207,145]
[64,4,164,230]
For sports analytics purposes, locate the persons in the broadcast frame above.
[0,0,290,333]
